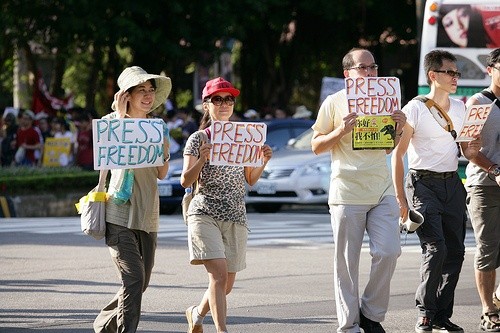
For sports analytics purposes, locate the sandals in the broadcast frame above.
[480,311,500,332]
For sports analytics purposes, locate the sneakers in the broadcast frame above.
[432,318,464,333]
[415,317,432,333]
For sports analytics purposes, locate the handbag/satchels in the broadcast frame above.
[75,185,107,240]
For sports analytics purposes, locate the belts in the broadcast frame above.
[421,172,453,180]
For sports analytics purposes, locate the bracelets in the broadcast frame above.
[163,155,170,163]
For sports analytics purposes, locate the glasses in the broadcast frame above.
[344,64,379,71]
[208,96,235,106]
[427,69,462,79]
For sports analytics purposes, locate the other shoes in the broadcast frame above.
[186,305,203,333]
[359,308,385,333]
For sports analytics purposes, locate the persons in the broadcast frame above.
[93,66,170,333]
[310,47,408,333]
[180,77,273,333]
[437,4,488,48]
[0,102,313,168]
[391,50,483,332]
[469,47,500,332]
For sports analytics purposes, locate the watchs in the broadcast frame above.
[493,167,500,176]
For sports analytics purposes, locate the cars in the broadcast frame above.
[155,119,408,214]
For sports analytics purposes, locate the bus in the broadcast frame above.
[418,0,500,185]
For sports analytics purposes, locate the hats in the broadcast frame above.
[111,66,172,114]
[202,77,240,103]
